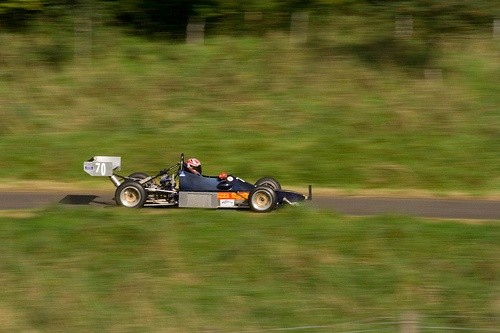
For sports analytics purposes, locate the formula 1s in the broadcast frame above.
[84,152,312,212]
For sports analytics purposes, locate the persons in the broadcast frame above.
[185,158,203,175]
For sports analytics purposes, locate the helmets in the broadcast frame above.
[186,158,202,175]
